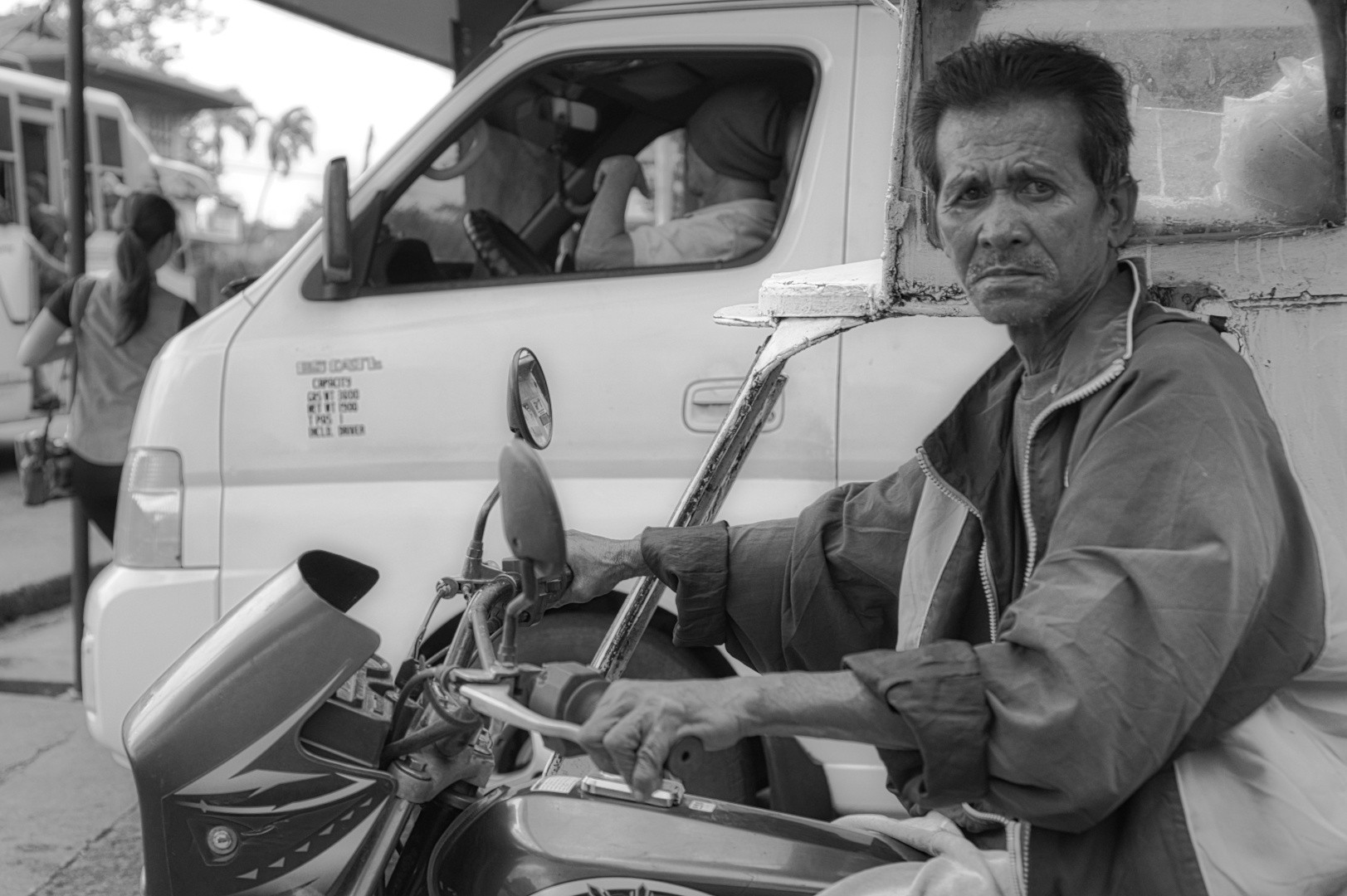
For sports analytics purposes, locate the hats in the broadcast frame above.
[682,79,792,183]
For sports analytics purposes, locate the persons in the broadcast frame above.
[504,27,1346,896]
[571,80,794,275]
[20,189,212,544]
[22,175,73,410]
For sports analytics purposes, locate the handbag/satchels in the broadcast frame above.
[18,435,78,503]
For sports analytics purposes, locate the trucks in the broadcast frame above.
[83,0,1346,819]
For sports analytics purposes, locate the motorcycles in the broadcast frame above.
[121,347,935,896]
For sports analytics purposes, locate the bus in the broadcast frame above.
[0,65,199,452]
